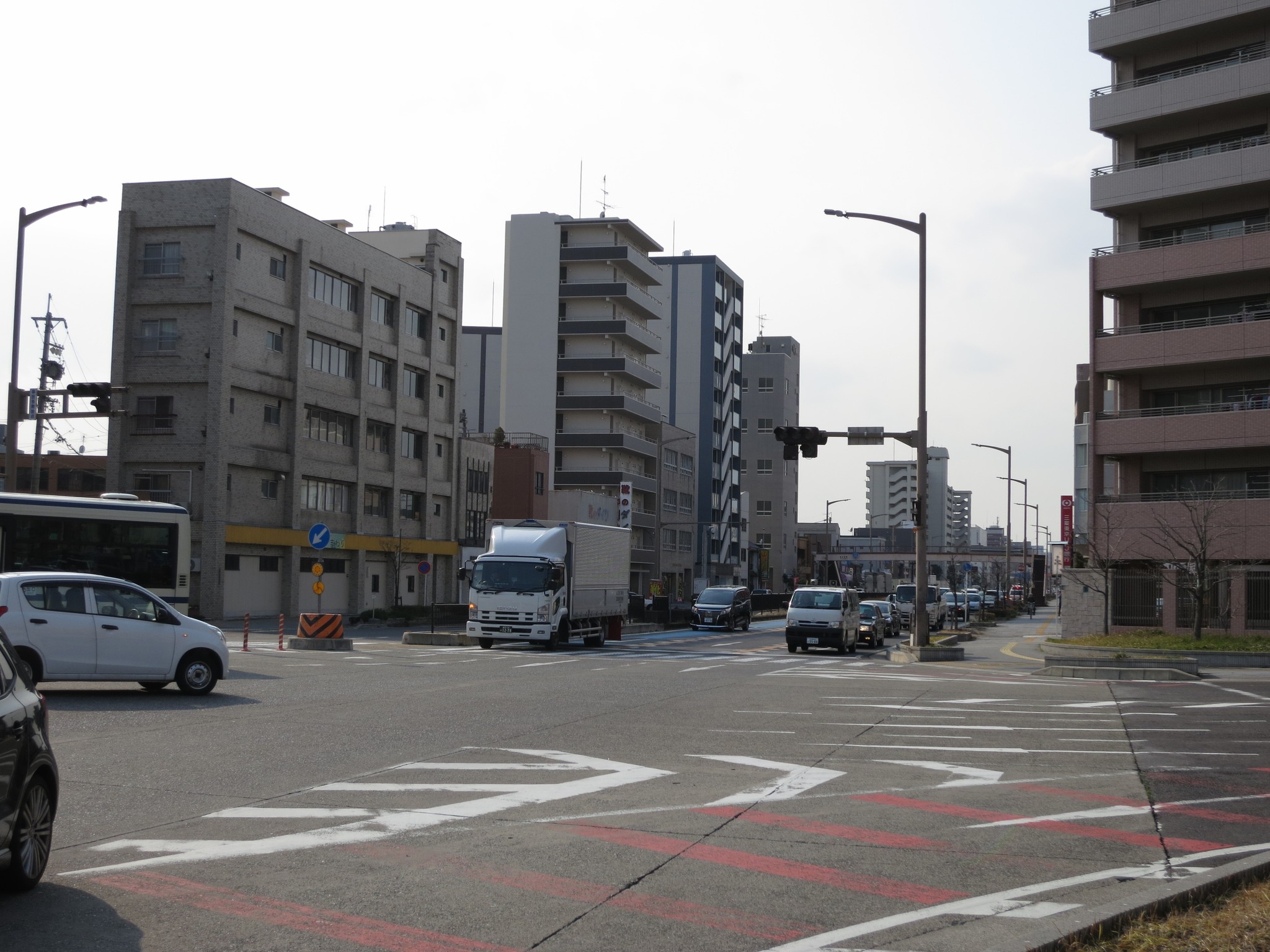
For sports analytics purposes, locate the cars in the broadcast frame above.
[627,591,653,611]
[751,589,772,594]
[0,626,60,895]
[856,602,886,649]
[860,600,901,638]
[0,570,229,697]
[940,585,1024,610]
[885,594,896,603]
[945,592,970,622]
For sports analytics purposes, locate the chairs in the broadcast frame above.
[829,594,841,608]
[46,588,87,614]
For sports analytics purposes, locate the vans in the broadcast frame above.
[689,585,753,632]
[966,593,983,615]
[782,586,861,654]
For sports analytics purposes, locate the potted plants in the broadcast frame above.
[503,441,511,449]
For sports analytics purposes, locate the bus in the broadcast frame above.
[0,492,191,617]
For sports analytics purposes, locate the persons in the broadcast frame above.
[1026,593,1036,615]
[1058,591,1061,616]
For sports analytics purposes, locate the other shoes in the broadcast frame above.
[1033,614,1035,615]
[1027,613,1029,615]
[1058,615,1060,616]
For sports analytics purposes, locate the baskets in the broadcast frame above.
[1028,604,1034,608]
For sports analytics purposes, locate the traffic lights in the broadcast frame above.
[91,398,111,413]
[66,382,112,398]
[774,426,820,442]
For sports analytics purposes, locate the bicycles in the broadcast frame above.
[1027,602,1035,619]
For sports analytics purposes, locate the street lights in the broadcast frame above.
[1035,531,1051,594]
[824,498,851,586]
[870,514,889,572]
[891,523,911,574]
[971,443,1012,601]
[1030,524,1048,595]
[4,195,107,493]
[823,207,930,647]
[996,476,1027,602]
[653,435,697,580]
[1013,503,1039,555]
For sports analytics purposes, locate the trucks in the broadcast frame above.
[456,519,633,652]
[895,584,949,632]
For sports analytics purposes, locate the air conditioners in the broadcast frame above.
[190,558,202,572]
